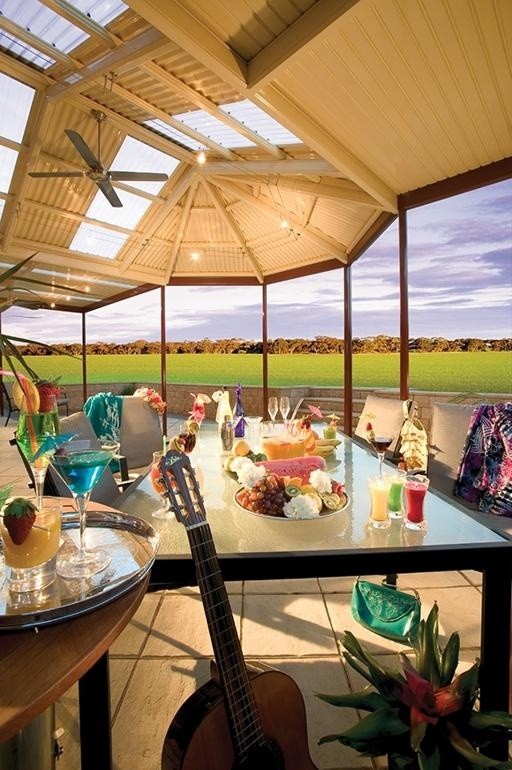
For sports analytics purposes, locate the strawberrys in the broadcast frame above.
[36,379,61,413]
[366,422,372,431]
[4,497,35,544]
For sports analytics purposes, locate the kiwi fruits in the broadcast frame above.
[284,487,300,499]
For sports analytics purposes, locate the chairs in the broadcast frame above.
[9,413,121,508]
[355,395,416,463]
[84,388,171,492]
[2,380,69,426]
[427,402,511,536]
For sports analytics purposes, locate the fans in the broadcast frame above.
[27,110,168,208]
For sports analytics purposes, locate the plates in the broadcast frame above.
[233,479,351,521]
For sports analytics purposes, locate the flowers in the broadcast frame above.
[311,599,512,770]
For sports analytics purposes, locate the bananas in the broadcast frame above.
[307,439,341,457]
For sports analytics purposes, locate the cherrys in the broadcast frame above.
[179,435,189,444]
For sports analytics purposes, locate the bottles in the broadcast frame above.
[215,383,245,452]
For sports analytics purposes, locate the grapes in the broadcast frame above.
[250,476,287,516]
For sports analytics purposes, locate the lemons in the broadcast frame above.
[11,379,40,415]
[174,435,186,452]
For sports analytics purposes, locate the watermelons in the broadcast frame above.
[255,456,327,485]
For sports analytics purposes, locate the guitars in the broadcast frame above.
[160,451,316,770]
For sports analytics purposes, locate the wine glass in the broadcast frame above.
[268,396,290,433]
[244,416,263,442]
[16,394,62,508]
[43,438,121,578]
[369,434,393,474]
[151,392,206,519]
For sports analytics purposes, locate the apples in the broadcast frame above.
[224,440,267,472]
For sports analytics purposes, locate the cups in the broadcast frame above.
[366,468,428,530]
[322,425,337,439]
[0,496,64,594]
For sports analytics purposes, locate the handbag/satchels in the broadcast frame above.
[351,582,421,643]
